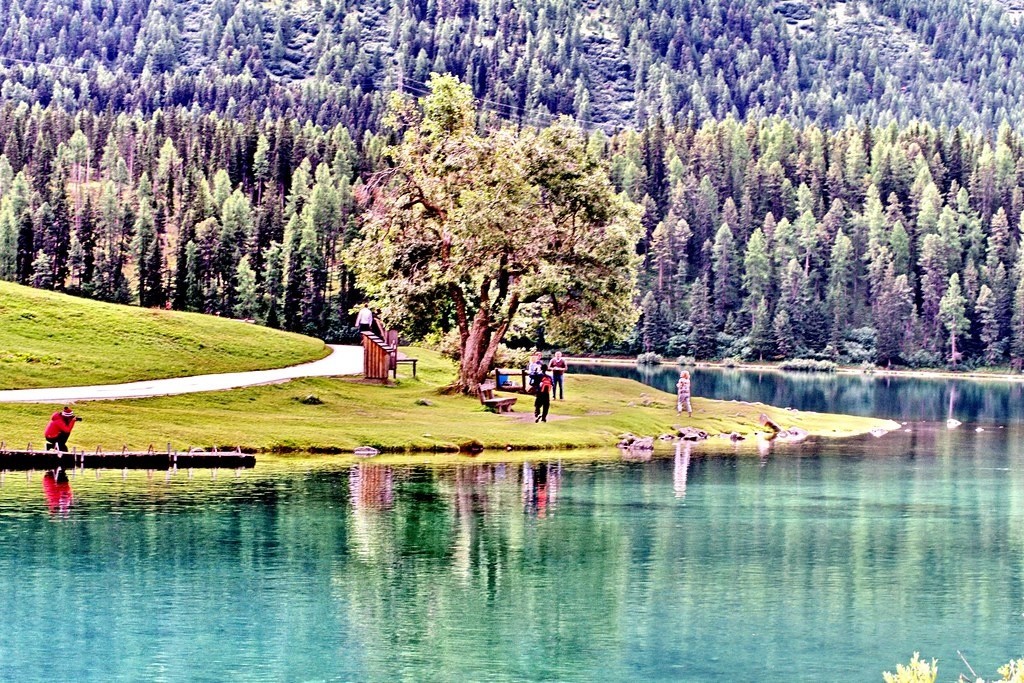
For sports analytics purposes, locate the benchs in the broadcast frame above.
[478,379,518,413]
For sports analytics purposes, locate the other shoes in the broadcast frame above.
[45,442,50,451]
[551,397,556,401]
[541,418,547,423]
[534,414,542,423]
[55,442,62,451]
[559,399,565,402]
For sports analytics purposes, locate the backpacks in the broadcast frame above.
[539,374,551,393]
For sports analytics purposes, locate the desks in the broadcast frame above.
[491,369,539,394]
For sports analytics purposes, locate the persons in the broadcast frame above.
[675,371,693,418]
[532,364,555,424]
[43,406,76,453]
[527,351,568,404]
[42,469,73,520]
[355,303,373,346]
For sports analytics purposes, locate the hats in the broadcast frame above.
[62,406,74,417]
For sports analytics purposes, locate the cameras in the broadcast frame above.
[72,416,82,421]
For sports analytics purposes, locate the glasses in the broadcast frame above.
[537,354,543,357]
